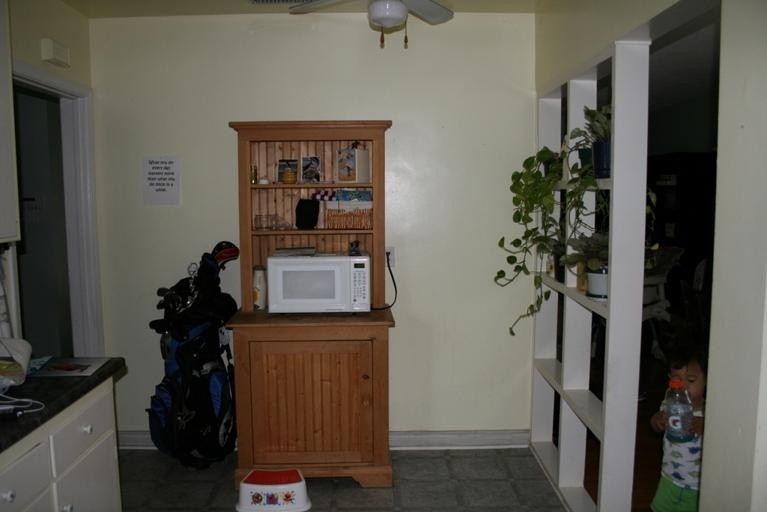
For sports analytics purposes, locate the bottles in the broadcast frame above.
[252,265,266,312]
[663,377,692,443]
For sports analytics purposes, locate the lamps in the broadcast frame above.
[365,3,414,50]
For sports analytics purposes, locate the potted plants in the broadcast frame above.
[565,223,609,297]
[586,104,612,179]
[494,144,565,289]
[562,129,593,177]
[491,226,564,338]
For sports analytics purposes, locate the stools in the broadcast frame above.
[230,468,314,511]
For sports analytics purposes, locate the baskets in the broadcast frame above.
[325,201,373,230]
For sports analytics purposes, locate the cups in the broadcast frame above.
[250,164,257,185]
[254,215,272,230]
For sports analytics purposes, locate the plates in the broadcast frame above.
[277,171,296,184]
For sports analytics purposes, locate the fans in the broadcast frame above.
[257,0,452,31]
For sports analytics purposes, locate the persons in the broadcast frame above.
[648,339,707,512]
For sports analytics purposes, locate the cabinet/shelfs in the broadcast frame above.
[0,355,128,511]
[522,29,654,512]
[220,117,399,488]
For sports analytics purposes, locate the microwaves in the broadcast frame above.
[265,249,372,315]
